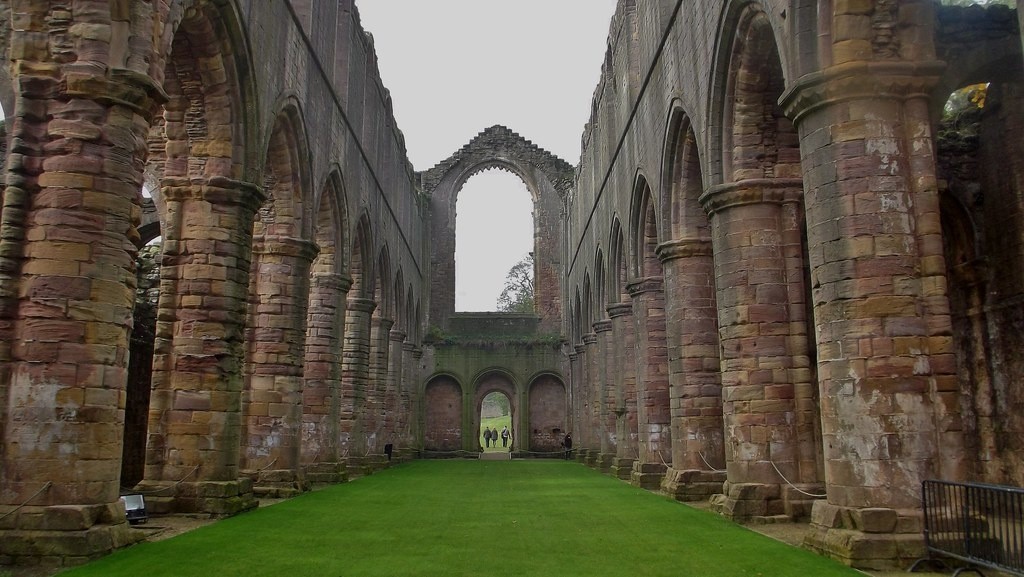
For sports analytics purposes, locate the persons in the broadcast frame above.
[564,431,572,459]
[491,427,498,447]
[483,426,492,448]
[501,426,511,447]
